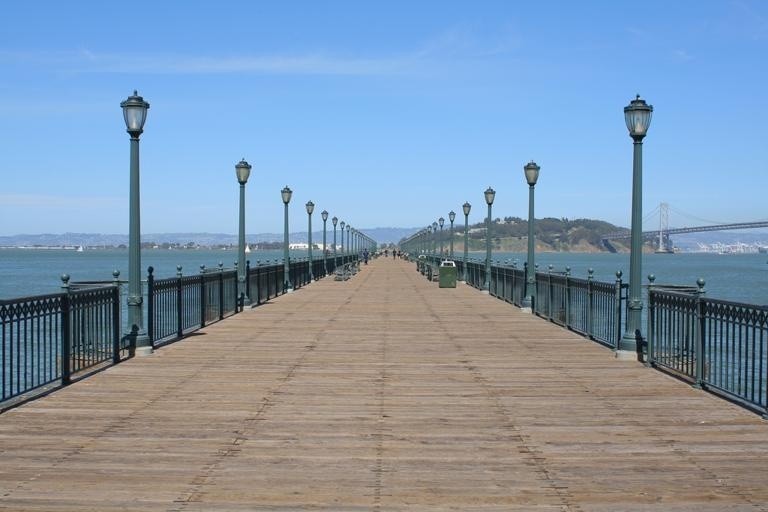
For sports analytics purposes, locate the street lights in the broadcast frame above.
[520,160,540,309]
[481,185,495,290]
[280,184,291,289]
[232,156,252,307]
[398,209,456,265]
[118,89,153,349]
[461,200,473,284]
[320,210,376,277]
[619,93,652,354]
[304,200,315,279]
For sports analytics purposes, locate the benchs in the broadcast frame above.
[400,253,439,282]
[333,252,380,281]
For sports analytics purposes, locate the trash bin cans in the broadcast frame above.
[400,252,404,259]
[418,255,427,265]
[368,252,372,259]
[375,252,379,257]
[438,258,457,288]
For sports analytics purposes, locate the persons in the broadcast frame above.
[371,248,401,259]
[363,248,369,264]
